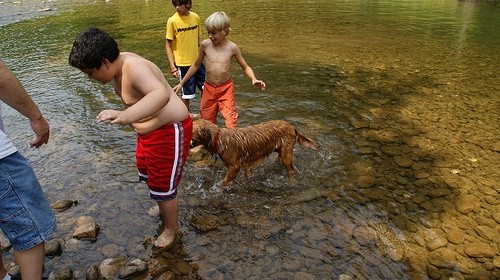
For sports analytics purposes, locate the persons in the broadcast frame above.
[68,28,193,248]
[173,10,266,129]
[165,0,206,110]
[0,57,57,280]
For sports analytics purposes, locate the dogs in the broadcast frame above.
[188,118,319,192]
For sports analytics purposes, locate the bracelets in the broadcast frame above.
[172,70,178,73]
